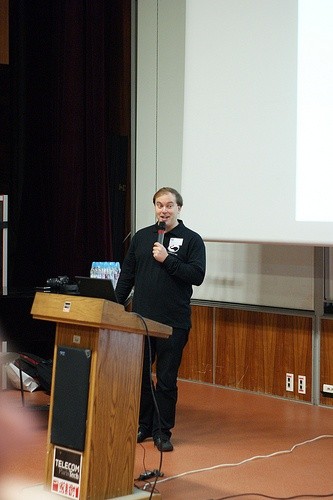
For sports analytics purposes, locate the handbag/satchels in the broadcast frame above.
[2,363,40,393]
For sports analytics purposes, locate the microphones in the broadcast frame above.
[157,221,166,245]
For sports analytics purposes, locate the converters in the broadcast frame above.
[137,471,155,481]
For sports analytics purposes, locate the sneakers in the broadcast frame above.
[137,428,151,443]
[154,433,174,452]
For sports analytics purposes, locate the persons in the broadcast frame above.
[115,187,206,452]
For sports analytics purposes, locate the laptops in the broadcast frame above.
[75,276,118,303]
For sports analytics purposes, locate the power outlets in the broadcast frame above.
[298,375,306,394]
[322,384,333,394]
[285,373,294,392]
